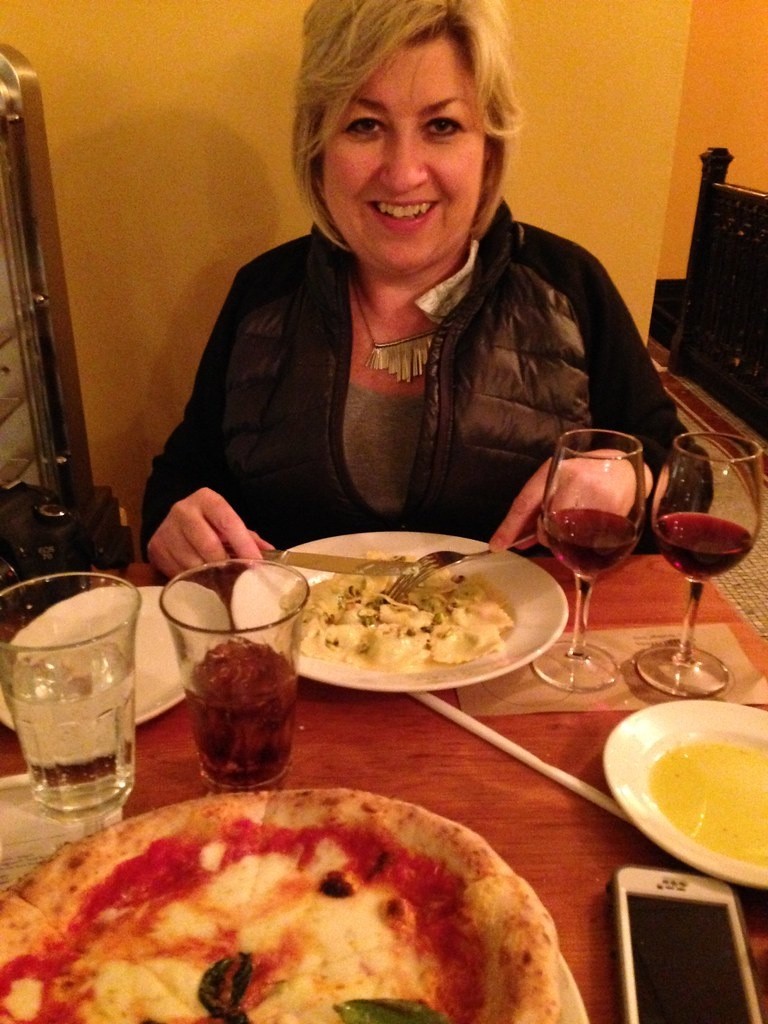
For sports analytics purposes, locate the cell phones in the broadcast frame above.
[613,866,763,1024]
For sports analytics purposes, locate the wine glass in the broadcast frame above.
[532,428,646,693]
[636,432,765,700]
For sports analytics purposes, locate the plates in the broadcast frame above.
[230,531,568,693]
[0,581,231,733]
[556,954,591,1024]
[601,698,768,889]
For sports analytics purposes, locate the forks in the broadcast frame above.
[385,530,535,603]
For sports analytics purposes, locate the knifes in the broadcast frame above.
[227,548,423,577]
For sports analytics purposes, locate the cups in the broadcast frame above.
[0,572,143,825]
[159,558,311,793]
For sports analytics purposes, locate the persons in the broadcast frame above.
[141,0,712,581]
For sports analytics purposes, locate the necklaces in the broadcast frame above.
[353,275,451,383]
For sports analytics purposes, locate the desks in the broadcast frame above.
[0,553,768,1024]
